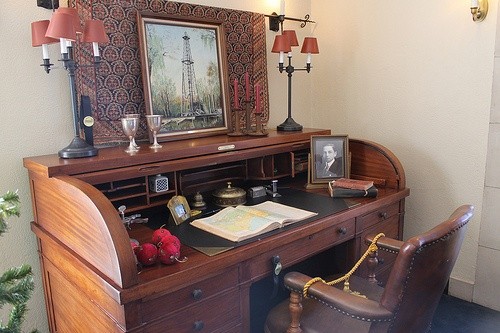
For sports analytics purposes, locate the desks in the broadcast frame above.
[36,175,411,333]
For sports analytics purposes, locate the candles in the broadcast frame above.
[234,78,239,107]
[255,84,261,113]
[245,72,251,100]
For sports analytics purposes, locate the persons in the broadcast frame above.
[316,144,342,177]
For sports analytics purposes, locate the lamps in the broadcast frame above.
[271,30,318,132]
[31,7,107,159]
[269,0,285,32]
[470,0,488,22]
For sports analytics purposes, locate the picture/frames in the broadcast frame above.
[307,135,351,190]
[136,11,228,142]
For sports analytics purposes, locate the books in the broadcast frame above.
[190,201,318,242]
[328,178,378,198]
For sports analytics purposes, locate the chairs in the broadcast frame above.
[262,204,475,333]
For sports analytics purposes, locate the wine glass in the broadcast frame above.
[123,114,141,149]
[121,118,139,153]
[146,115,163,148]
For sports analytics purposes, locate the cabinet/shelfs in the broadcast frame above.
[132,265,250,333]
[352,201,404,267]
